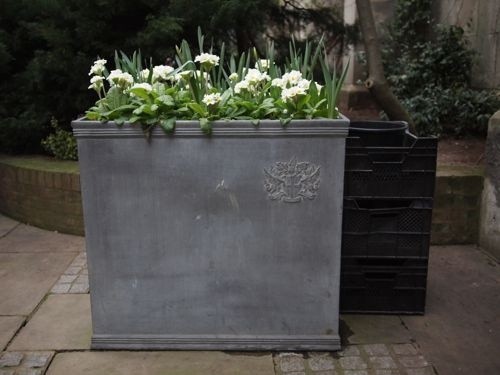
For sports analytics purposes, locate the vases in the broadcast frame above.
[69,110,352,353]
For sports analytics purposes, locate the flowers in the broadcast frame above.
[82,30,345,136]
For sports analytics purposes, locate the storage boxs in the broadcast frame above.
[339,118,439,317]
